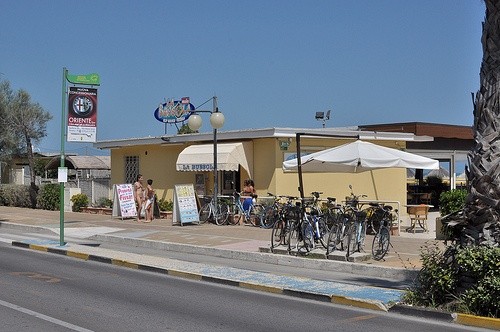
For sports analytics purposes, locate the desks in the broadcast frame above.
[402,205,434,232]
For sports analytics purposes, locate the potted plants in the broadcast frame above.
[436,189,467,241]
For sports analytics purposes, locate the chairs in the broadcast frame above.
[408,205,429,234]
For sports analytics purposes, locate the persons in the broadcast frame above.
[134,174,153,223]
[239,180,256,225]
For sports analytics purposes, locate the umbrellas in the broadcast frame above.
[282,133,440,239]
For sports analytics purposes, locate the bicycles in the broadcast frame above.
[198,180,266,228]
[260,184,399,261]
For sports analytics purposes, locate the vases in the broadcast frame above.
[72,206,83,212]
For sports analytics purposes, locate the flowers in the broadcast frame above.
[71,193,89,207]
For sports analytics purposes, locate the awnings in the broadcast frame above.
[176,142,251,179]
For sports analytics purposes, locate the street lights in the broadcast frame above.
[187,95,224,213]
